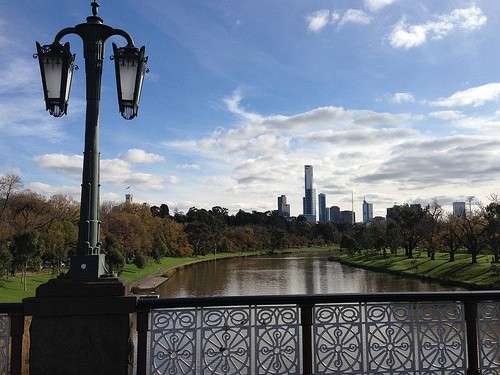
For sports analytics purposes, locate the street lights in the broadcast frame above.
[33,0,147,297]
